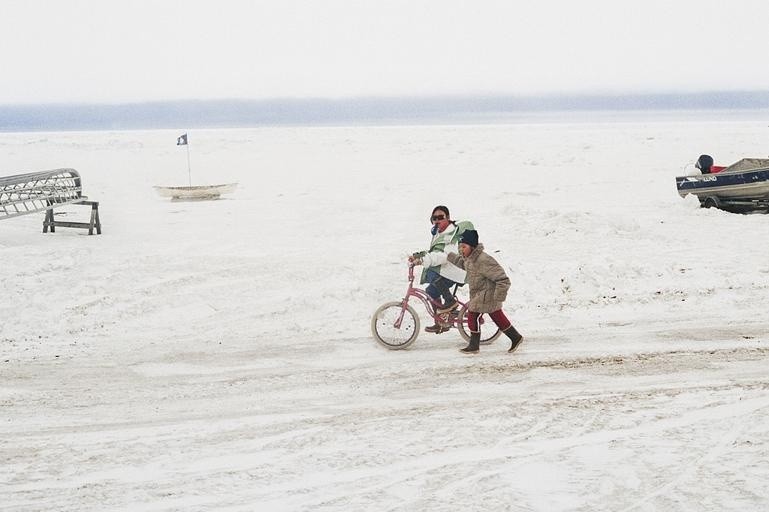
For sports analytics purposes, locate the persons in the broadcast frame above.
[443,228,524,354]
[408,204,474,334]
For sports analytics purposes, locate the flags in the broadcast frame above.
[177,134,188,147]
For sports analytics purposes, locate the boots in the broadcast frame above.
[425,298,449,332]
[503,324,523,352]
[460,331,480,353]
[433,276,458,313]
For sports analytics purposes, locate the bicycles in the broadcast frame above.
[371,251,502,352]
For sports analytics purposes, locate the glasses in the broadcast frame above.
[432,215,444,220]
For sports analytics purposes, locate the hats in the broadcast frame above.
[458,229,478,247]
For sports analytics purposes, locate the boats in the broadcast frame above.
[672,154,768,217]
[151,179,242,198]
